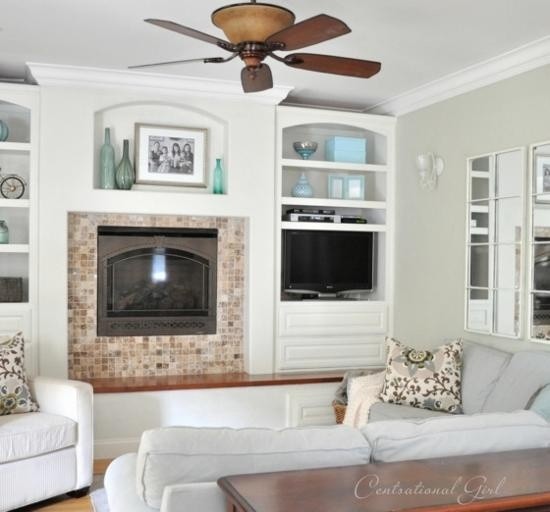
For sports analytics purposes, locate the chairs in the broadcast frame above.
[0,335,94,512]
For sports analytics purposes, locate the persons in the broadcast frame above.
[150,142,162,170]
[179,144,193,175]
[170,143,181,171]
[157,144,170,174]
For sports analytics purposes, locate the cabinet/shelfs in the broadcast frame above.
[0,82,37,375]
[467,154,497,332]
[276,106,395,372]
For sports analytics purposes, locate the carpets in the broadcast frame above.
[90,474,109,512]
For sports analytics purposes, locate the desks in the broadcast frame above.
[216,449,550,512]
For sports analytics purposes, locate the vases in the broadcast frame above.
[116,139,135,190]
[99,128,116,190]
[213,159,223,194]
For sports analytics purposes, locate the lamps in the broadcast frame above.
[416,153,444,191]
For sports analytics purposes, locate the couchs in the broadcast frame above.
[104,330,550,512]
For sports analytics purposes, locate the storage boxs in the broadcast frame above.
[325,136,366,164]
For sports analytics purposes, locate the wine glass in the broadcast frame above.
[293,142,319,159]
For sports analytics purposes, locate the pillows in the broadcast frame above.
[0,330,39,416]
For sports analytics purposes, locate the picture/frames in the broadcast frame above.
[536,155,550,203]
[135,122,206,188]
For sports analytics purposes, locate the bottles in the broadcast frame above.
[213,157,224,195]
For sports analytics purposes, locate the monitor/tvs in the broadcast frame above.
[280,230,379,302]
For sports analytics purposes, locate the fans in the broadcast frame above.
[129,0,381,93]
